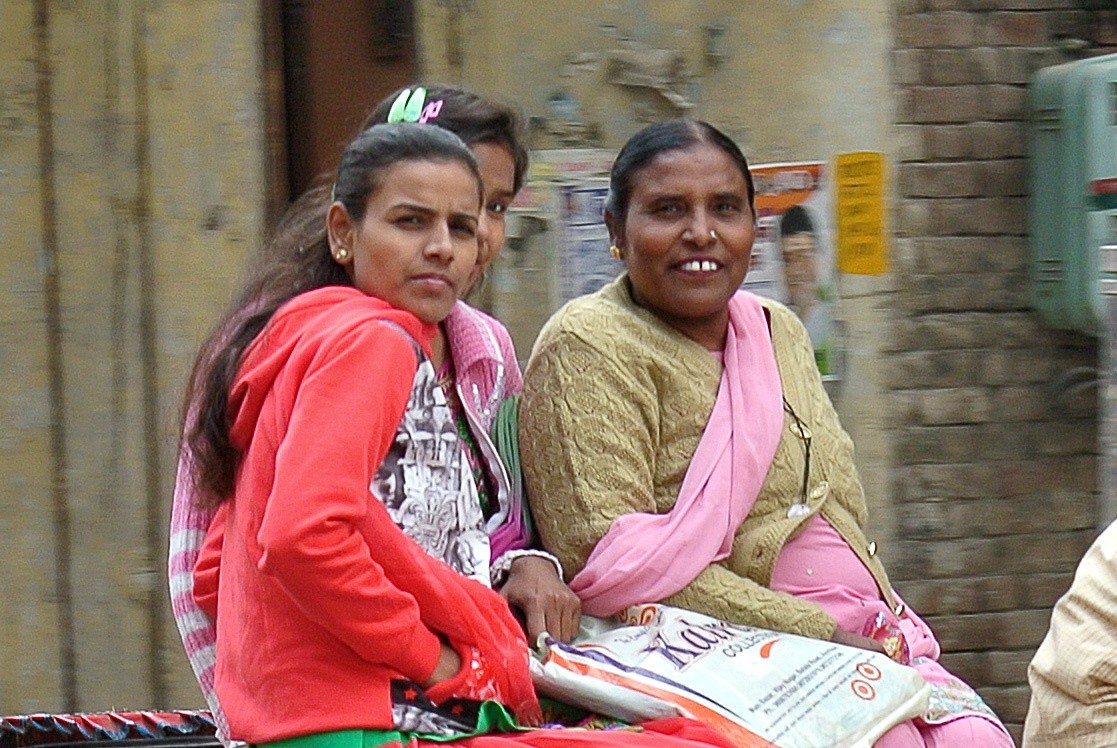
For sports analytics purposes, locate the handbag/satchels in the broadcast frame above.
[525,601,933,748]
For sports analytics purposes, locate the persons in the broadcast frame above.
[168,85,740,748]
[1021,518,1117,748]
[779,204,834,351]
[520,120,1018,748]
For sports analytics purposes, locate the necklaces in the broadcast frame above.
[762,305,812,519]
